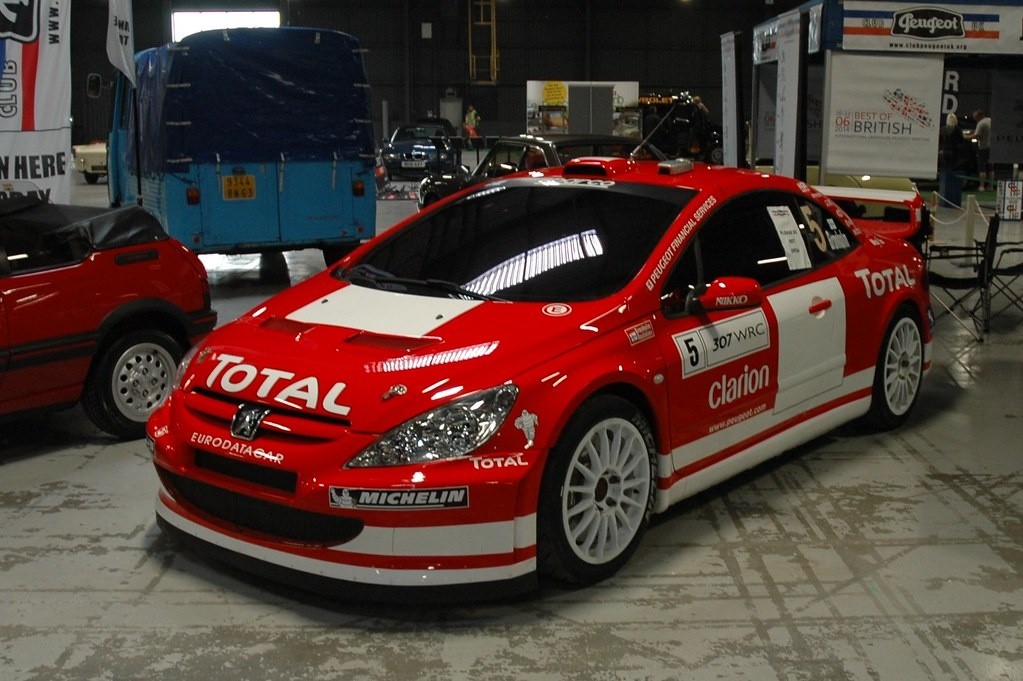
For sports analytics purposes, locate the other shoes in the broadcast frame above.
[986,186,994,192]
[977,186,985,191]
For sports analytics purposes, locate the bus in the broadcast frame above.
[85,26,378,268]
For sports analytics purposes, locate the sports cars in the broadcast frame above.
[146,159,939,594]
[0,179,218,440]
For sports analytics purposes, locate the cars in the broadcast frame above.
[381,116,461,181]
[417,131,669,215]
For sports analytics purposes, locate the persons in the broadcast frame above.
[464,105,480,137]
[543,112,554,127]
[940,113,966,173]
[963,109,995,191]
